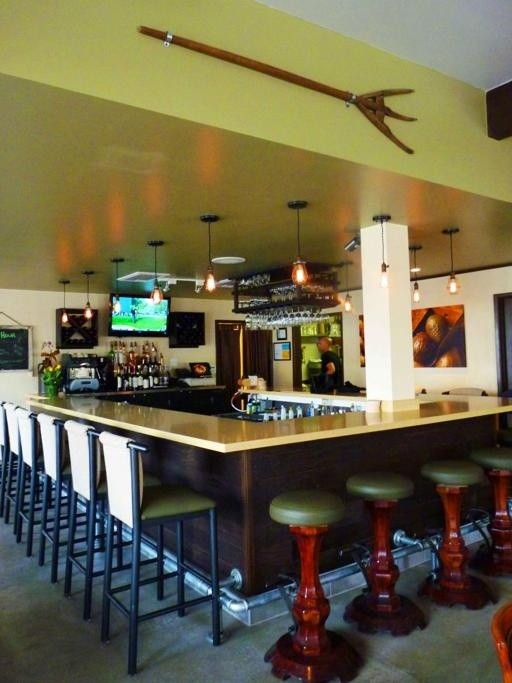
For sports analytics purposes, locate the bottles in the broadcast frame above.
[262,399,356,422]
[107,340,169,390]
[245,399,260,415]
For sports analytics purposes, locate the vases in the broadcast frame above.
[45,384,57,404]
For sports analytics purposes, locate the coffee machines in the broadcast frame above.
[60,354,111,392]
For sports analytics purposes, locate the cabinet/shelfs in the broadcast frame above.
[169,312,205,348]
[56,308,98,349]
[65,384,232,416]
[227,259,344,314]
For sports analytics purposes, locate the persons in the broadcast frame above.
[130,307,137,323]
[316,336,341,392]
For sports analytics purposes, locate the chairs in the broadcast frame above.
[442,388,488,396]
[415,385,426,394]
[490,602,512,683]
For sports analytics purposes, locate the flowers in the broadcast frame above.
[41,364,64,400]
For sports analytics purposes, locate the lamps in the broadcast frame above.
[335,261,353,313]
[147,240,163,306]
[59,280,70,324]
[288,200,308,288]
[410,246,422,302]
[110,259,124,315]
[442,229,461,295]
[372,215,391,289]
[200,216,219,293]
[82,271,94,319]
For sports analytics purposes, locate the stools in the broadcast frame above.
[467,445,512,575]
[343,470,427,637]
[418,458,497,610]
[263,487,365,683]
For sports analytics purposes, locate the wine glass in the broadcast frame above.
[239,272,331,332]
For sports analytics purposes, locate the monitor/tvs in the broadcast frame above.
[108,294,171,338]
